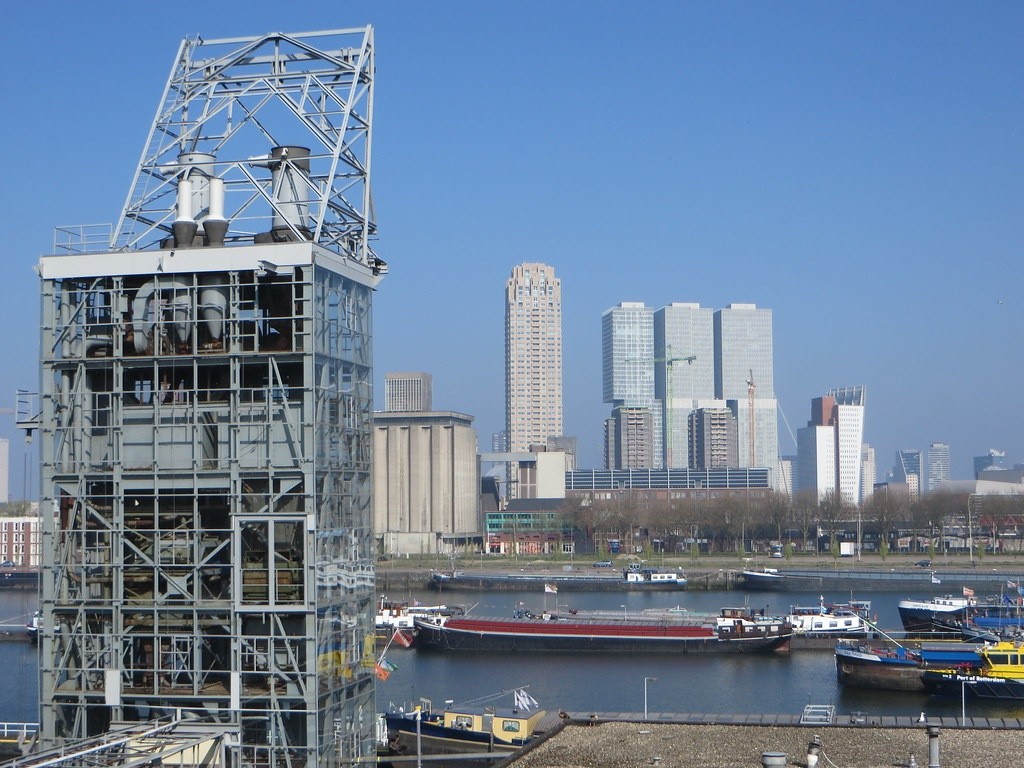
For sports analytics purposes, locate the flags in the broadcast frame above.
[378,657,399,672]
[963,586,974,596]
[413,599,421,606]
[520,689,539,708]
[374,664,390,682]
[930,574,941,584]
[1016,585,1024,596]
[393,629,413,648]
[514,691,530,712]
[1007,580,1018,590]
[544,583,558,594]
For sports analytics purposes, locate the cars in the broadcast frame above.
[915,560,932,567]
[593,561,612,567]
[918,633,1024,700]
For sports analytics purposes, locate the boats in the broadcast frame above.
[413,599,800,652]
[372,594,466,645]
[832,625,1024,691]
[619,562,689,591]
[780,589,879,651]
[743,566,784,583]
[898,592,974,636]
[377,684,546,768]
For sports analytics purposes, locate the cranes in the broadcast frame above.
[624,345,697,467]
[745,371,762,466]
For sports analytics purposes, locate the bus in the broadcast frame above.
[772,545,784,558]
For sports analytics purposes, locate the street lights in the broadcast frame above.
[967,493,983,562]
